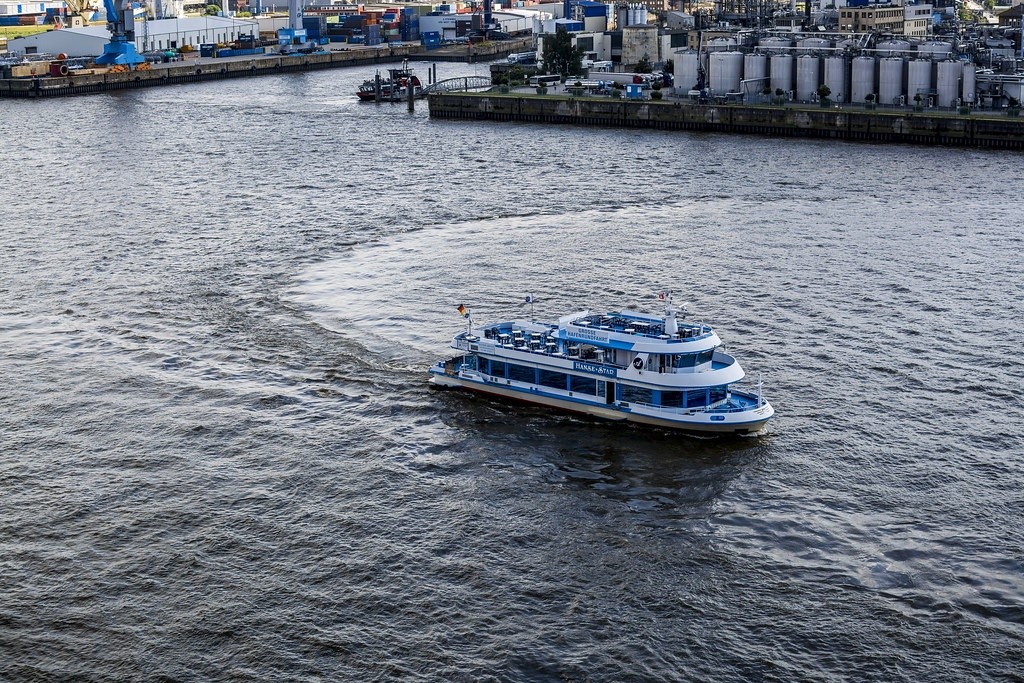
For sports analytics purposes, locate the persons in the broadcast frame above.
[553,82,556,89]
[683,330,686,338]
[679,330,683,337]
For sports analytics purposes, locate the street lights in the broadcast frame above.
[956,78,961,112]
[718,58,723,95]
[927,98,930,110]
[810,71,813,102]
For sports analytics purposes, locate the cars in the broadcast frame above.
[639,73,664,81]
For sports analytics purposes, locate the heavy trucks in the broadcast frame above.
[588,72,643,85]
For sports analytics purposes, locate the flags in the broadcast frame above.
[458,305,469,319]
[659,294,664,299]
[525,295,532,303]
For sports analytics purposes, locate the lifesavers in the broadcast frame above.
[634,358,643,369]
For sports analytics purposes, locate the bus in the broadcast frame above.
[528,74,562,88]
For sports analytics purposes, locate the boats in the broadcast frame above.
[430,291,774,436]
[355,57,422,103]
